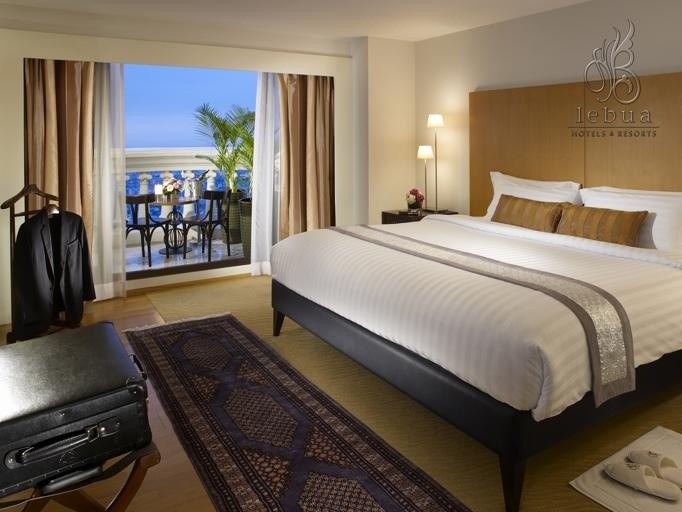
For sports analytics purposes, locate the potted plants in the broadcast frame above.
[194,103,255,244]
[226,110,256,259]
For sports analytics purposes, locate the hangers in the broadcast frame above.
[18,205,83,234]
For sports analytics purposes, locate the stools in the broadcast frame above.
[0,443,161,512]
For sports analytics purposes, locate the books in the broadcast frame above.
[398,209,422,215]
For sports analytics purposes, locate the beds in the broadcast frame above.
[271,172,682,512]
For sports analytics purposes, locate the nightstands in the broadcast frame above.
[382,210,458,224]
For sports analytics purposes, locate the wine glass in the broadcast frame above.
[178,181,186,200]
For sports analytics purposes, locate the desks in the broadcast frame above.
[150,198,199,255]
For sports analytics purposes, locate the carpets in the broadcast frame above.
[569,426,681,512]
[123,312,473,512]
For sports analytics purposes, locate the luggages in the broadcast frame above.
[0,318,156,499]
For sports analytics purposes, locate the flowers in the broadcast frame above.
[163,178,184,196]
[406,189,424,209]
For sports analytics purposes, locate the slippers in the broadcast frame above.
[602,459,682,502]
[626,447,682,489]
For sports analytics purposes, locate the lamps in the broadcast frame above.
[427,114,445,213]
[417,145,434,208]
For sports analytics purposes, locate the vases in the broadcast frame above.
[163,194,178,200]
[408,206,421,216]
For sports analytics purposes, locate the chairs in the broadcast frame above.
[126,193,171,267]
[180,190,232,263]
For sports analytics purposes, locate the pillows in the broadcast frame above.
[491,194,571,233]
[483,172,582,221]
[555,203,648,247]
[580,186,682,256]
